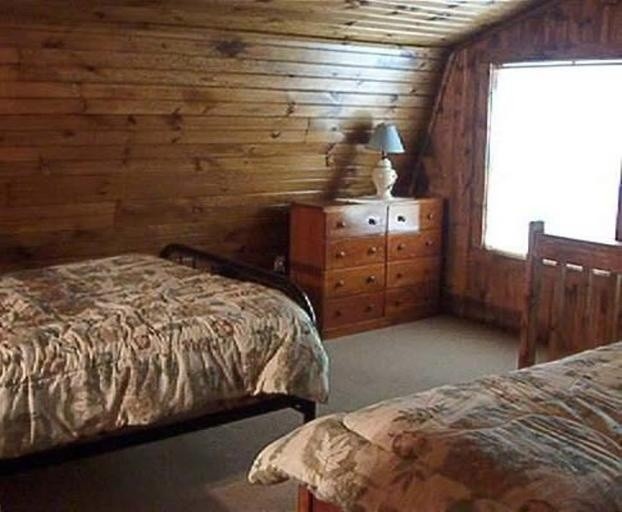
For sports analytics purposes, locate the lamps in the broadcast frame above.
[364,123,406,200]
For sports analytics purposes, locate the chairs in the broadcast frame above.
[518,222,621,367]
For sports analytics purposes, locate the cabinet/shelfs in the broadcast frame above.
[283,197,448,342]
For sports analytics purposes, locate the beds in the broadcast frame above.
[296,340,622,512]
[1,239,320,489]
